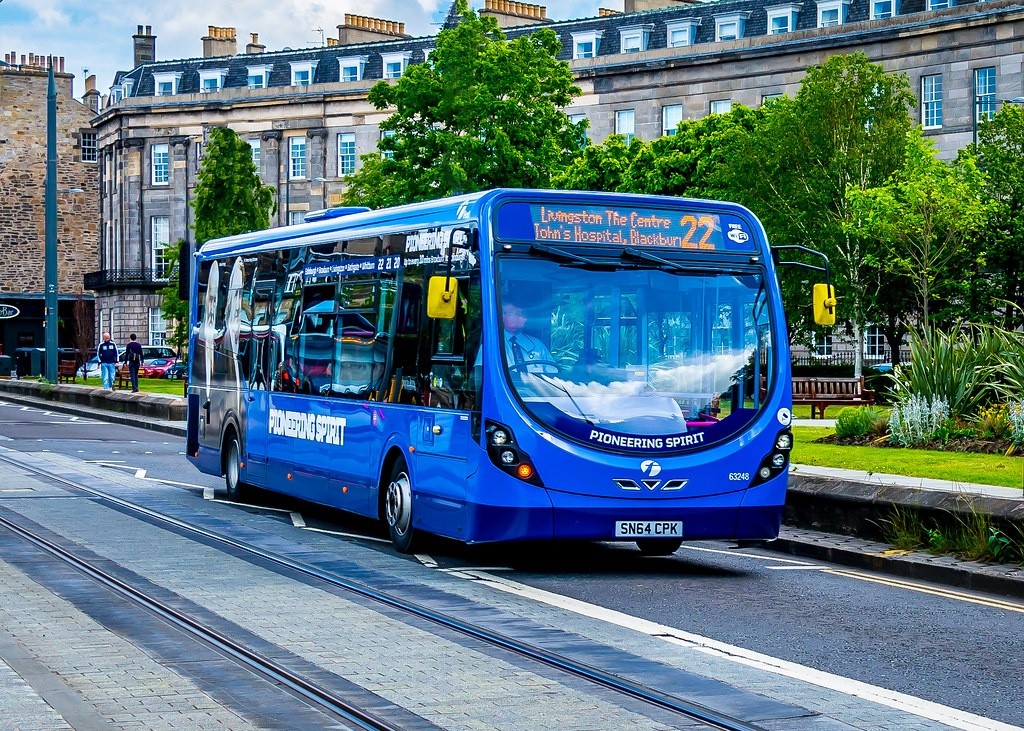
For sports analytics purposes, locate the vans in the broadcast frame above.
[74,344,183,380]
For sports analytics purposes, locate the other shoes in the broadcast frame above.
[131,390,140,393]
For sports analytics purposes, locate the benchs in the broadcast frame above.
[58,360,77,384]
[182,375,189,398]
[760,373,877,419]
[113,363,131,390]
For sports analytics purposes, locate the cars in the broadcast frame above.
[142,357,186,380]
[162,361,190,379]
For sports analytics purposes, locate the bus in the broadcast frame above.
[181,186,839,556]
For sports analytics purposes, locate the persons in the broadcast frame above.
[194,260,219,399]
[474,294,560,392]
[214,257,247,381]
[123,333,144,393]
[97,332,120,390]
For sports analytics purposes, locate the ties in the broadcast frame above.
[509,335,528,374]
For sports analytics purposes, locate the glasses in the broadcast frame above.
[505,309,524,317]
